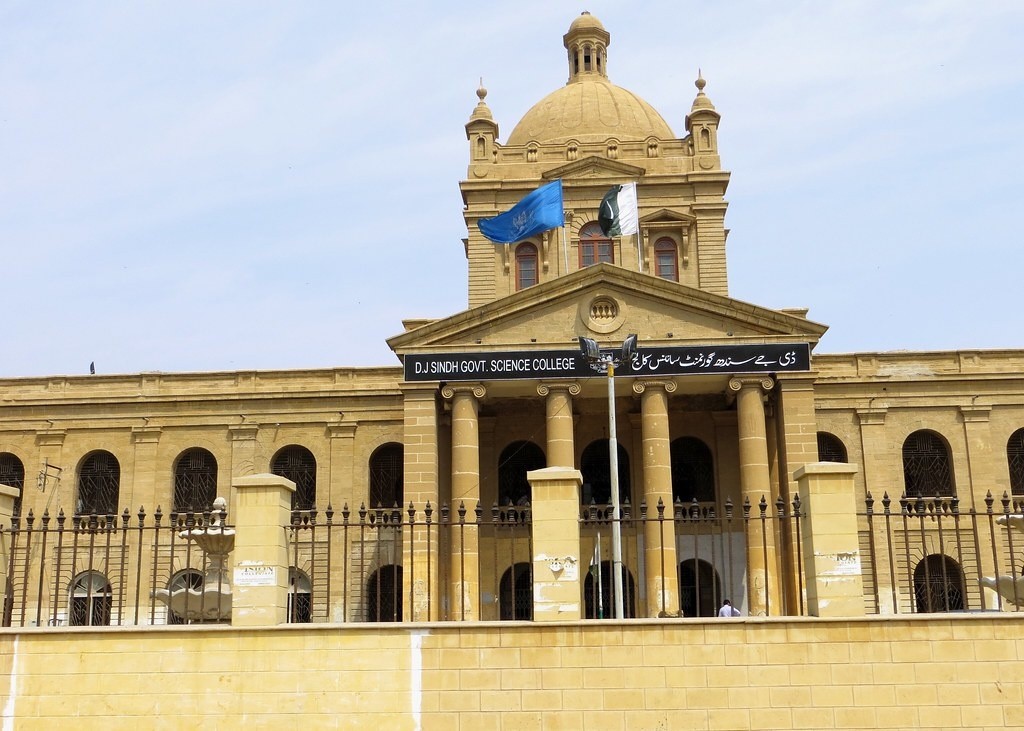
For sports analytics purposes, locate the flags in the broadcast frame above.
[588,542,598,583]
[598,181,638,238]
[477,179,564,242]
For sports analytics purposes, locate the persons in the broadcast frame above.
[718,600,740,617]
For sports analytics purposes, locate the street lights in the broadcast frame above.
[577,333,638,619]
